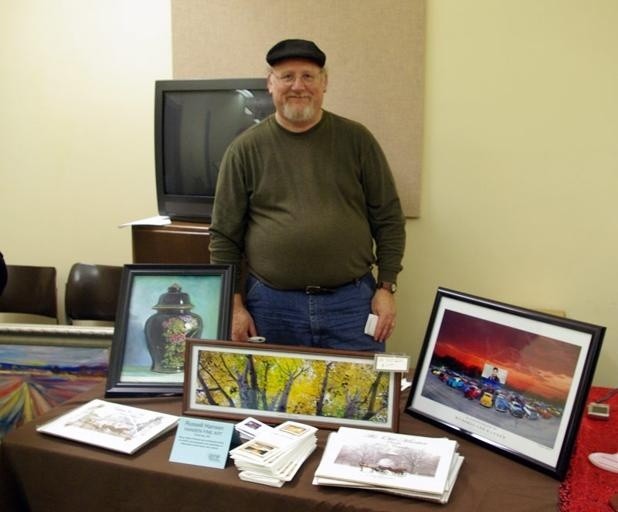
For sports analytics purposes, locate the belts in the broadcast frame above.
[298,286,335,295]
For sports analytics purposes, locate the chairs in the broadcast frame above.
[65,264,124,326]
[1,265,61,324]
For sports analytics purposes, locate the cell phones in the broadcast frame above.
[586,400,611,420]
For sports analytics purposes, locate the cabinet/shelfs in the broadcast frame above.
[131,220,211,265]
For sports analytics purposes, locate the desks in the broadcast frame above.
[1,367,618,512]
[0,331,111,445]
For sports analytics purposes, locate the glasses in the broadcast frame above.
[270,71,318,82]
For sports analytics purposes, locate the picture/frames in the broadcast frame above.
[104,265,235,400]
[182,338,401,434]
[405,286,606,480]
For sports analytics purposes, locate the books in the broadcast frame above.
[38,397,180,455]
[230,418,466,503]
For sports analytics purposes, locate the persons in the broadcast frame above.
[488,366,501,383]
[208,38,407,352]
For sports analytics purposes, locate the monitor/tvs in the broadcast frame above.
[152,78,278,225]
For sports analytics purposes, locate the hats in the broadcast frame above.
[266,40,325,68]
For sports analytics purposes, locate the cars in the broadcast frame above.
[433,366,561,420]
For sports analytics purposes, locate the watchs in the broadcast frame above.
[375,280,399,295]
